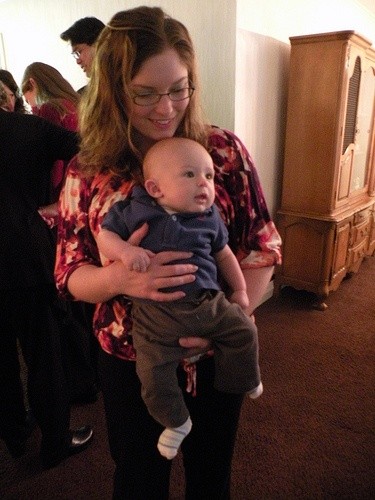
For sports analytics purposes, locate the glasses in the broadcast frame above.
[72,50,84,60]
[120,80,195,106]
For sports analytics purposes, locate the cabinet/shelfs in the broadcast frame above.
[273,30,375,310]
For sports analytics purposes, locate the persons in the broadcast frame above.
[23,63,101,405]
[0,69,24,114]
[60,16,105,79]
[54,8,283,500]
[97,137,264,460]
[0,110,93,468]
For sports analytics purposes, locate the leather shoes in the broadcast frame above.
[63,425,94,454]
[9,408,35,457]
